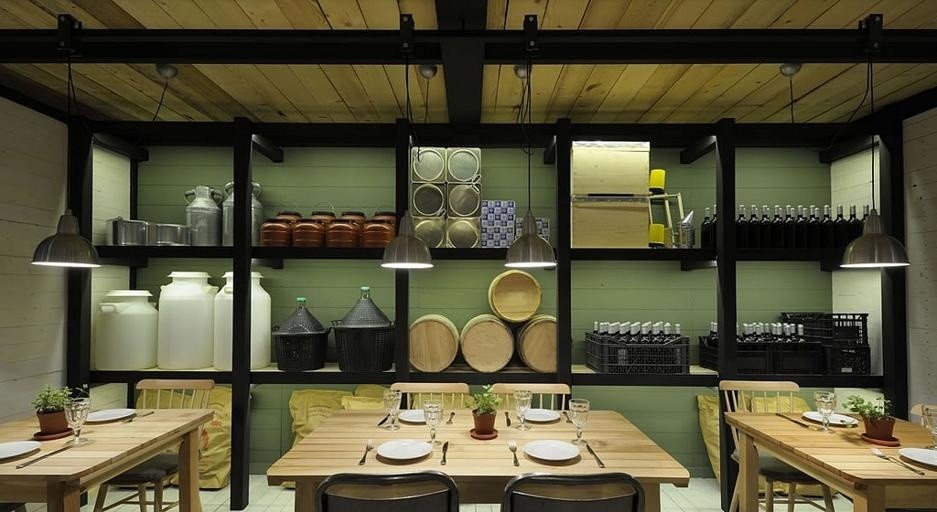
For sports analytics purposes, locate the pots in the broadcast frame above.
[103,217,192,246]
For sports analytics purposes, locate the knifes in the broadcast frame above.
[586,443,604,469]
[15,445,72,469]
[377,413,390,427]
[505,411,512,428]
[440,441,449,465]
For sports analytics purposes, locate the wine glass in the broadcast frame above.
[569,398,591,444]
[513,389,531,430]
[813,389,836,433]
[919,403,936,447]
[424,400,441,447]
[63,398,90,449]
[383,389,401,430]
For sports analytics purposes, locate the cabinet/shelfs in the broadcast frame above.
[64,111,907,510]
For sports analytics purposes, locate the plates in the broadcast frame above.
[411,148,481,250]
[802,411,860,427]
[83,408,137,423]
[523,439,581,463]
[523,408,560,422]
[377,439,433,460]
[898,446,937,473]
[398,409,428,423]
[405,273,562,374]
[1,440,42,459]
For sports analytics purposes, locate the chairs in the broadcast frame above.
[719,380,834,512]
[389,383,571,410]
[94,378,215,512]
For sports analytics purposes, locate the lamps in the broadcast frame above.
[381,13,434,270]
[502,15,557,268]
[836,13,911,269]
[28,12,103,271]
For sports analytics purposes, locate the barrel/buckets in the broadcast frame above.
[488,269,543,324]
[96,290,157,372]
[459,312,518,374]
[213,272,272,370]
[157,271,217,372]
[408,313,460,372]
[517,313,557,372]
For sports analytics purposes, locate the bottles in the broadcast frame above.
[591,317,683,374]
[706,319,806,367]
[701,205,871,259]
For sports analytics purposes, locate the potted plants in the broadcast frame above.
[465,384,503,439]
[843,394,900,446]
[30,384,89,441]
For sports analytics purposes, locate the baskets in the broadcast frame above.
[699,312,871,376]
[584,332,690,376]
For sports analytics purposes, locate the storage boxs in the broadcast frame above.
[569,141,651,248]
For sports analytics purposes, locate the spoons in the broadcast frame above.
[870,446,923,475]
[774,413,824,432]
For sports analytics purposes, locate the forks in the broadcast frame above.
[508,441,521,467]
[563,410,573,425]
[358,440,375,465]
[447,412,456,424]
